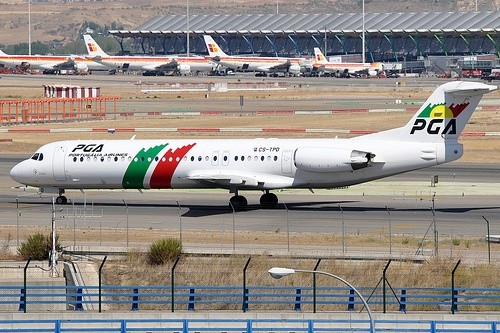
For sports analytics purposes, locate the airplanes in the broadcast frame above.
[9,78,500,211]
[0,33,384,77]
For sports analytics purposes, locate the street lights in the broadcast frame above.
[268,267,376,333]
